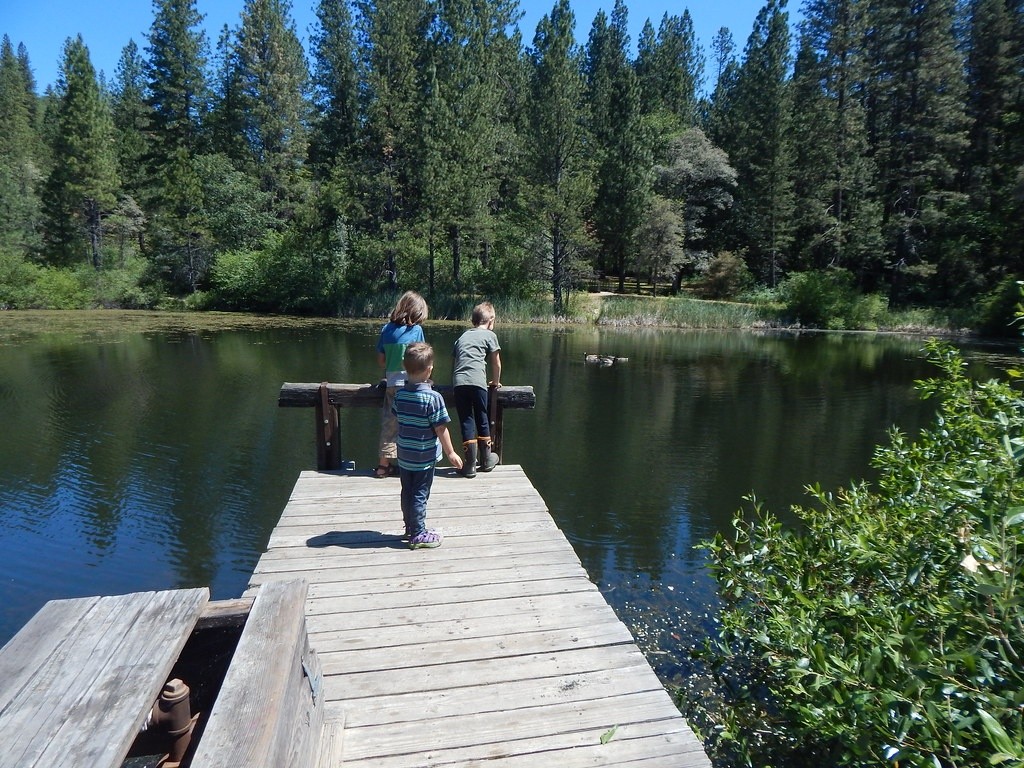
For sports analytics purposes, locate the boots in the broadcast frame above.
[462,439,478,477]
[478,436,499,472]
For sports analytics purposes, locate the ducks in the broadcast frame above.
[583,352,629,367]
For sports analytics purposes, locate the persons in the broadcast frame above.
[373,291,430,478]
[391,344,463,547]
[453,302,501,479]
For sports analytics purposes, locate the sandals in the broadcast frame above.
[376,463,400,478]
[405,531,442,549]
